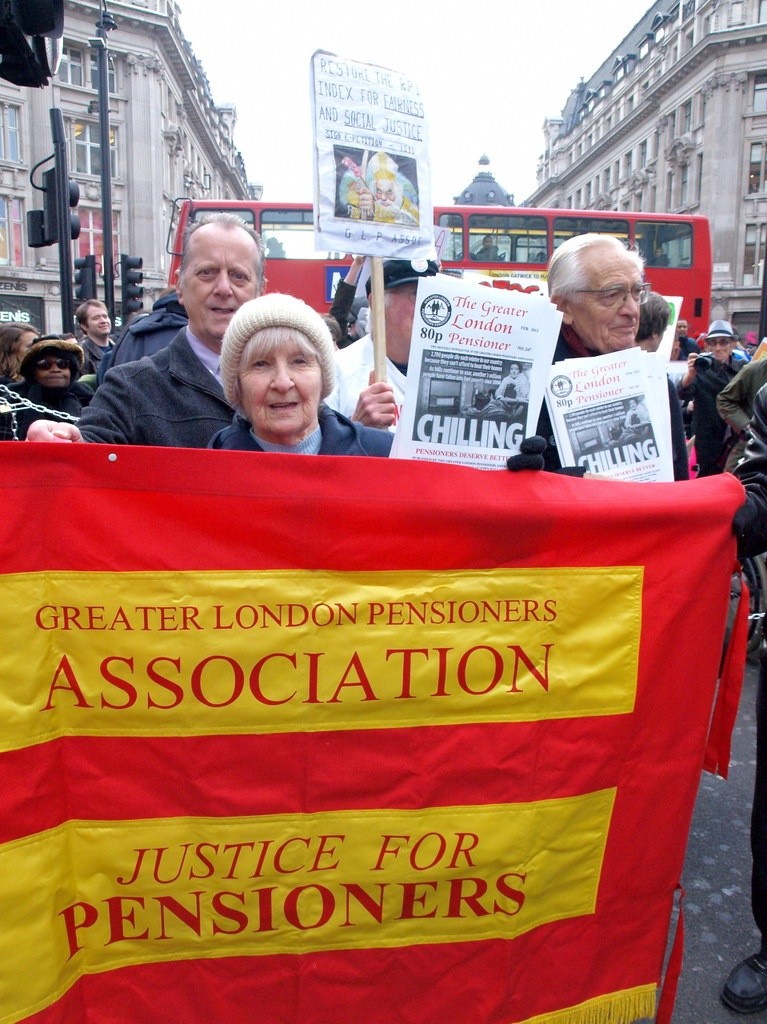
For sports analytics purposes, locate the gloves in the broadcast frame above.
[507,435,546,471]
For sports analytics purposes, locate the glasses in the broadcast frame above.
[709,339,731,347]
[577,283,651,308]
[36,358,70,371]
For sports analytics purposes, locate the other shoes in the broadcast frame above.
[720,954,767,1013]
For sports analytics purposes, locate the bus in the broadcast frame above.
[165,197,713,359]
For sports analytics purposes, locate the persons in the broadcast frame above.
[1,217,766,663]
[607,400,651,447]
[492,363,531,418]
[346,153,419,224]
[721,379,767,1012]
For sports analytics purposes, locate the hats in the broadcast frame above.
[19,334,85,382]
[703,320,740,343]
[219,293,335,405]
[365,259,439,299]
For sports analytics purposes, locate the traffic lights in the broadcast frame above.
[0,0,65,88]
[120,253,143,314]
[41,169,79,243]
[73,255,96,301]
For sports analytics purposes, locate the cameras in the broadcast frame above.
[693,355,716,373]
